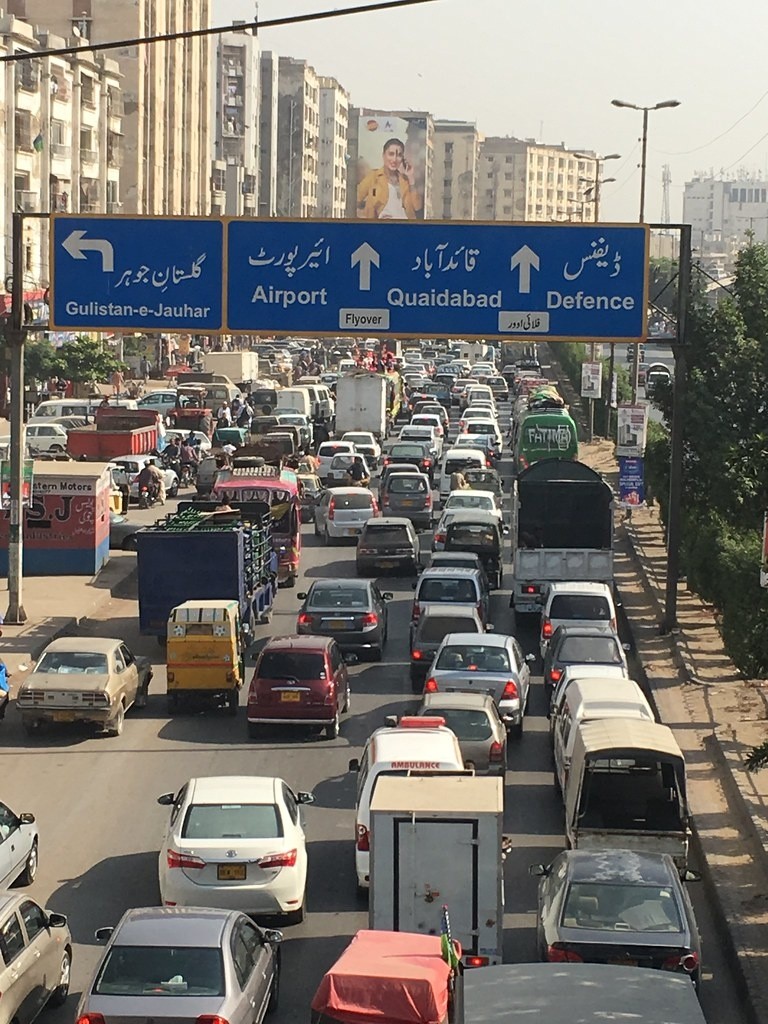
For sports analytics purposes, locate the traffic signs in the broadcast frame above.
[49,213,647,343]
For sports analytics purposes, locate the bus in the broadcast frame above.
[203,455,302,587]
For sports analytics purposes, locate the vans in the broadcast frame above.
[313,485,378,545]
[625,342,646,362]
[444,511,509,589]
[410,566,491,644]
[550,680,656,802]
[378,473,433,529]
[549,664,627,741]
[348,713,470,906]
[537,580,622,663]
[540,626,629,700]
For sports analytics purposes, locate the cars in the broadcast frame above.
[526,848,703,994]
[295,577,396,663]
[411,604,496,699]
[76,905,283,1024]
[245,632,353,741]
[0,796,38,902]
[433,512,455,552]
[17,636,154,739]
[414,690,514,782]
[420,633,536,737]
[1,337,580,518]
[151,774,318,925]
[629,363,649,386]
[440,490,505,529]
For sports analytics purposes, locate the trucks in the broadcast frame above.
[164,598,254,714]
[564,718,694,886]
[506,455,621,628]
[366,766,513,970]
[644,363,672,399]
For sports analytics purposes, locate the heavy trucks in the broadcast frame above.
[133,499,285,647]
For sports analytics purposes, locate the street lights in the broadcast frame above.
[611,98,683,226]
[554,154,623,223]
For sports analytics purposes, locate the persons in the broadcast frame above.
[139,355,153,385]
[245,393,255,409]
[217,401,232,427]
[180,441,200,475]
[300,450,319,473]
[232,394,245,416]
[55,378,67,400]
[347,457,368,488]
[138,458,164,503]
[184,431,201,452]
[112,369,124,396]
[117,463,133,515]
[356,138,423,220]
[217,413,228,428]
[449,464,466,489]
[158,438,181,479]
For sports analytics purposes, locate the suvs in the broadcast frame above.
[421,552,489,601]
[0,887,73,1024]
[355,514,421,576]
